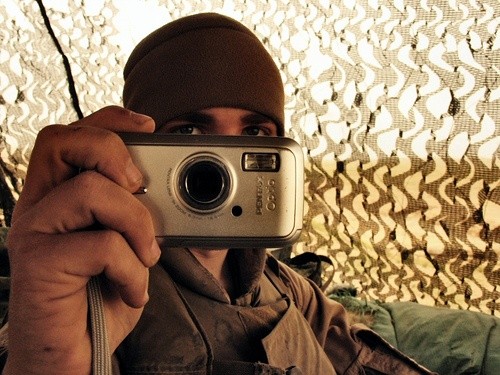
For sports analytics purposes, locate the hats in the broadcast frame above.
[122,12,285,137]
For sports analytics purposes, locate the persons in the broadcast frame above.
[3,12,440,375]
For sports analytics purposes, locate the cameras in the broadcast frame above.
[79,132,304,249]
